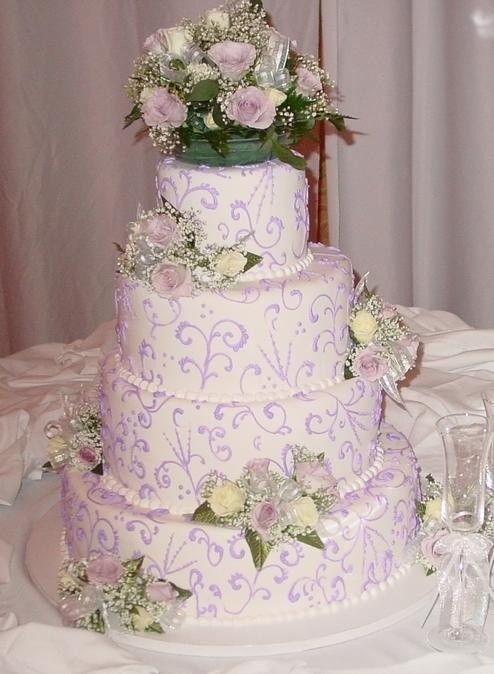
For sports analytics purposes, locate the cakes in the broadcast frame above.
[59,148,424,628]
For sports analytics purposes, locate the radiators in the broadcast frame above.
[344,271,421,409]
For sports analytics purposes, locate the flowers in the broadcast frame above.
[413,472,494,576]
[42,381,105,481]
[122,1,357,168]
[114,204,264,298]
[189,444,339,573]
[57,551,193,634]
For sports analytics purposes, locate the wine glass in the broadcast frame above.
[426,393,494,658]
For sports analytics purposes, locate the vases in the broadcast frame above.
[168,130,276,167]
